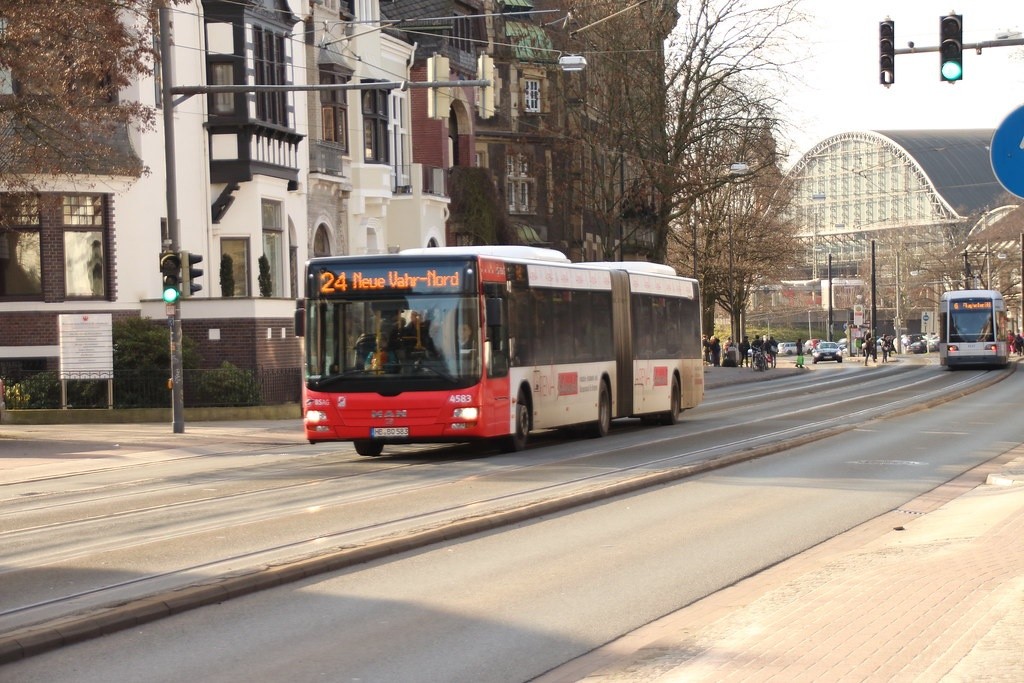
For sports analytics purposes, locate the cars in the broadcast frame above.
[805,338,849,356]
[901,334,939,353]
[813,342,844,364]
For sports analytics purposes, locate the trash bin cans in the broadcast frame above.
[728,347,740,367]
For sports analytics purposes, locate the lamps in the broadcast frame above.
[559,52,588,72]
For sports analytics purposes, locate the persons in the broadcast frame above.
[795,339,804,368]
[365,332,397,374]
[864,338,877,366]
[902,335,910,346]
[881,333,897,362]
[460,323,474,349]
[1008,330,1024,356]
[381,310,436,349]
[751,335,778,367]
[703,334,732,367]
[740,336,751,367]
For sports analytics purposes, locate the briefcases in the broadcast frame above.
[770,345,779,353]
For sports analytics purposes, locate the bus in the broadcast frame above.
[939,250,1009,369]
[293,244,706,455]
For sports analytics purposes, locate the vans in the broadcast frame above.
[778,342,811,356]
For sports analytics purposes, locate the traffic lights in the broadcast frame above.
[182,250,205,297]
[938,15,964,81]
[160,252,184,303]
[878,20,895,85]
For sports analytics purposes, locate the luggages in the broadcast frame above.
[796,356,805,365]
